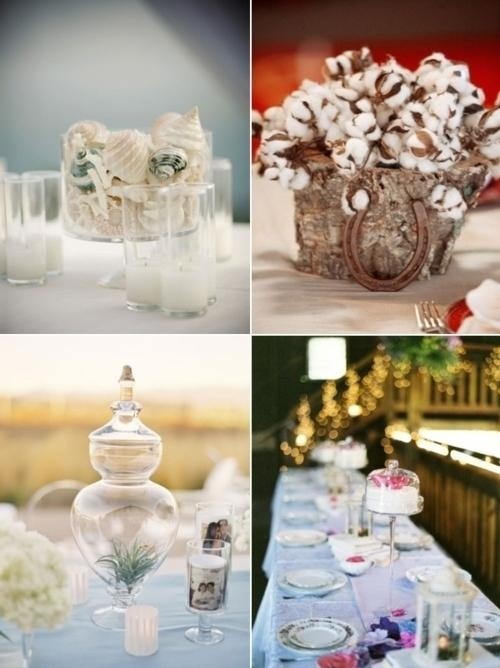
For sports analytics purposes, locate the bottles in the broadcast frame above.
[67,366,181,634]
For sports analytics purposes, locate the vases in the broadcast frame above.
[256,463,499,666]
[290,161,491,292]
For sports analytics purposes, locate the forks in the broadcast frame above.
[414,298,452,334]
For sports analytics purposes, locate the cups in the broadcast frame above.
[0,169,67,286]
[208,158,234,264]
[342,555,375,575]
[122,181,216,319]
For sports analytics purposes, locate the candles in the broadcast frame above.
[1,220,62,283]
[120,210,234,316]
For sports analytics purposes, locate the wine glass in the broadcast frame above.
[184,499,234,645]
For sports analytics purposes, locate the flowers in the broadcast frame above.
[250,43,500,189]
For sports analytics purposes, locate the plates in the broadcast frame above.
[405,565,472,588]
[279,617,359,655]
[282,474,326,525]
[377,529,434,550]
[277,567,348,597]
[439,606,500,648]
[275,529,327,547]
